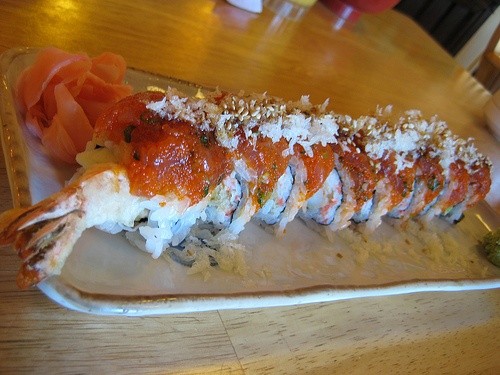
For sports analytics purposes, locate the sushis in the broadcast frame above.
[0,89,492,288]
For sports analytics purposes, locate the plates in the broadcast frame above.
[0,45,500,315]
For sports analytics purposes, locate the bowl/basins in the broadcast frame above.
[320,0,401,22]
